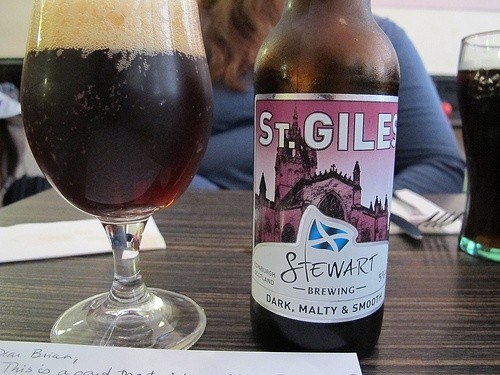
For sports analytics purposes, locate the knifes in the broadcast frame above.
[392,212,423,240]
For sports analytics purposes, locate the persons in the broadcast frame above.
[188,0,467,195]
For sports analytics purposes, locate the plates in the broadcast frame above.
[390,189,463,234]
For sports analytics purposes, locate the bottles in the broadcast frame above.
[251,0,401,355]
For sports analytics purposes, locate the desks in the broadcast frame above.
[0,188,500,375]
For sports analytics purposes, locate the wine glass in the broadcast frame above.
[20,0,214,350]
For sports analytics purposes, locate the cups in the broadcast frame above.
[458,29,500,262]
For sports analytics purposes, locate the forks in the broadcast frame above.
[415,210,463,230]
